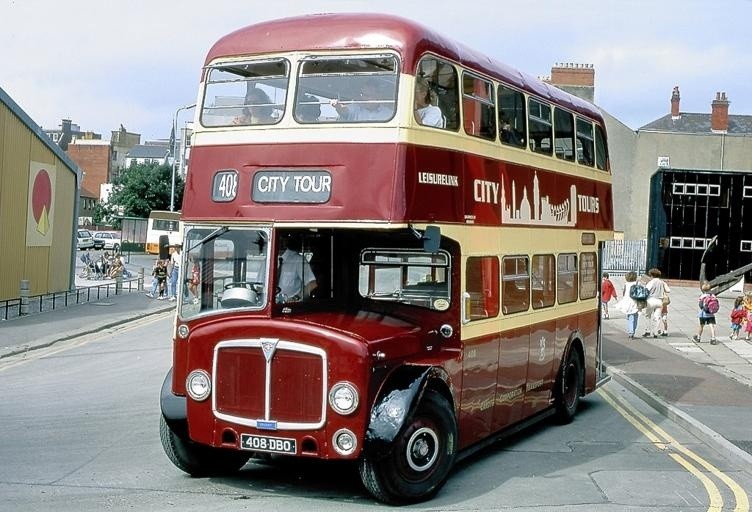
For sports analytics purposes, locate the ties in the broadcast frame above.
[277,258,283,283]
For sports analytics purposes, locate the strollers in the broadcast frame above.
[79,252,134,282]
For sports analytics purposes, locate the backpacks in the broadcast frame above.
[698,293,719,314]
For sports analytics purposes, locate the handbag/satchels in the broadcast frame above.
[663,293,669,306]
[630,285,648,300]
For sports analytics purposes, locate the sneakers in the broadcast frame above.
[642,330,667,338]
[146,293,177,301]
[693,335,700,342]
[710,339,716,345]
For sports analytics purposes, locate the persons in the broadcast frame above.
[255,231,318,304]
[96,244,200,302]
[601,266,752,345]
[231,70,453,130]
[489,111,515,144]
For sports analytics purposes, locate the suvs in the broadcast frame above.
[92,232,122,250]
[76,229,105,251]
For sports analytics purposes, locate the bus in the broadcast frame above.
[154,12,622,507]
[145,209,249,262]
